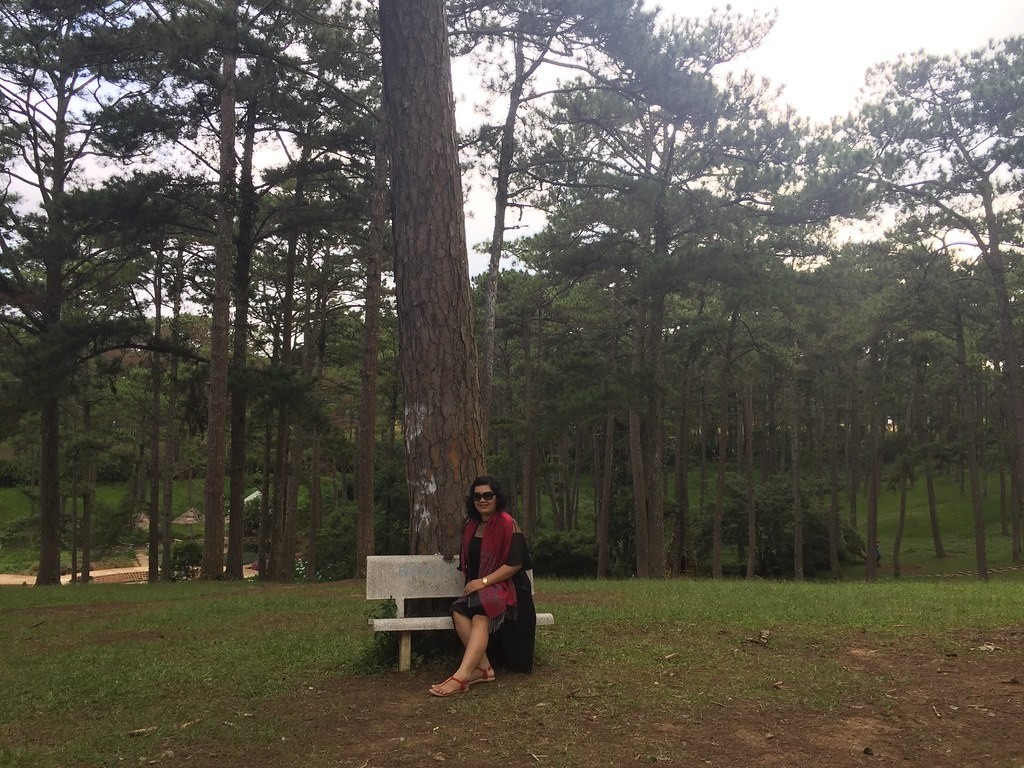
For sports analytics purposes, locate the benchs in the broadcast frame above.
[366,554,556,674]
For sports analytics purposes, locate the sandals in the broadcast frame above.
[428,676,469,696]
[469,665,495,684]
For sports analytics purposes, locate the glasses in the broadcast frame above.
[473,492,496,501]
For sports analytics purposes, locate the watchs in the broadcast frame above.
[482,577,488,586]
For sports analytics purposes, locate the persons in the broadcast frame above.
[430,474,537,697]
